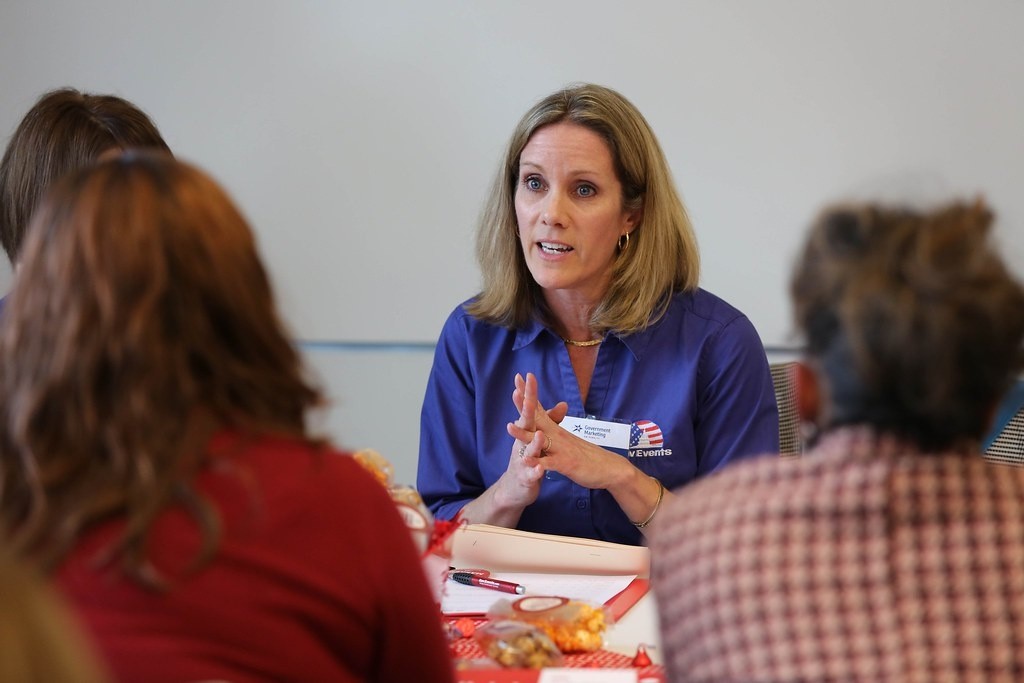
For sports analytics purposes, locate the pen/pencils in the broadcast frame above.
[446,565,527,596]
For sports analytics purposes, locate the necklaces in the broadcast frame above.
[568,337,604,348]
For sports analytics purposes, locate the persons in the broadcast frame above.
[1,149,454,683]
[421,84,779,549]
[1,90,175,276]
[652,201,1023,682]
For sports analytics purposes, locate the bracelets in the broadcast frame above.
[630,478,663,527]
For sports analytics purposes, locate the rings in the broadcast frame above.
[545,436,551,451]
[518,444,527,461]
